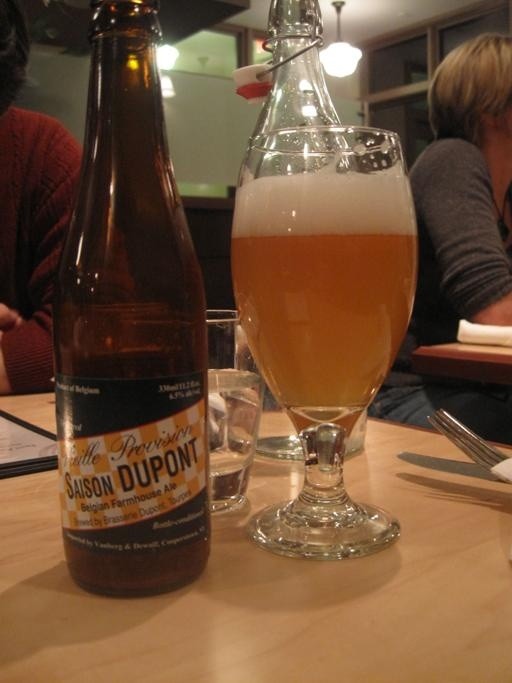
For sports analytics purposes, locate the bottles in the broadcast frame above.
[234,0,368,461]
[51,0,212,598]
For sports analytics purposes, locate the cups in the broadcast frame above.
[205,309,266,516]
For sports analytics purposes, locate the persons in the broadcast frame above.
[369,32,512,445]
[1,1,136,396]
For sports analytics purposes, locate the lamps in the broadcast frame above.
[320,2,361,77]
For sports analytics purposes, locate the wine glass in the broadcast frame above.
[230,125,418,559]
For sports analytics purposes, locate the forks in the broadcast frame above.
[427,407,512,473]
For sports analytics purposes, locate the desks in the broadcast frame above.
[0,391,512,683]
